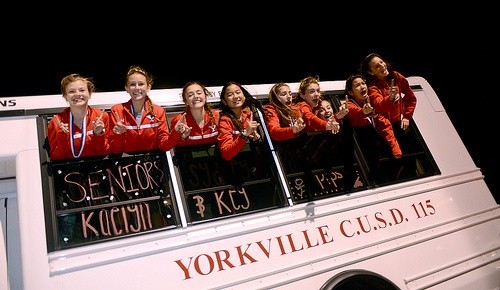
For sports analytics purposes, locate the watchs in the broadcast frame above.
[239,132,248,140]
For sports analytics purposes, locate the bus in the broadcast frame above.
[0,76,500,290]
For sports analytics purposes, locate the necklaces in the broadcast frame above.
[68,110,86,158]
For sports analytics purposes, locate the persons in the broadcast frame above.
[343,73,402,161]
[261,82,306,140]
[360,52,418,130]
[289,75,340,135]
[170,81,221,145]
[314,94,349,124]
[47,74,112,160]
[109,65,192,152]
[217,82,264,161]
